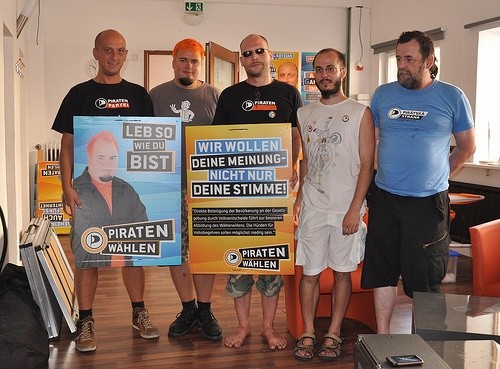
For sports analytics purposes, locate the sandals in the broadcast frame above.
[293,334,317,361]
[319,333,342,361]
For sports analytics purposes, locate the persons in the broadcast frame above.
[292,48,375,362]
[278,62,298,88]
[147,38,223,340]
[51,29,159,351]
[211,34,302,350]
[359,30,476,335]
[73,132,149,269]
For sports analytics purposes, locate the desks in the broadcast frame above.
[447,191,484,247]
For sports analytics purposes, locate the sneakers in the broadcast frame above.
[75,315,97,352]
[196,310,222,340]
[169,305,199,336]
[131,307,160,340]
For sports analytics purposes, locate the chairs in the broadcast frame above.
[282,209,379,339]
[469,219,500,299]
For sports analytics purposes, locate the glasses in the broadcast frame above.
[241,48,268,58]
[313,67,343,76]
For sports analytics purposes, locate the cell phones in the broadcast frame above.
[386,354,424,367]
